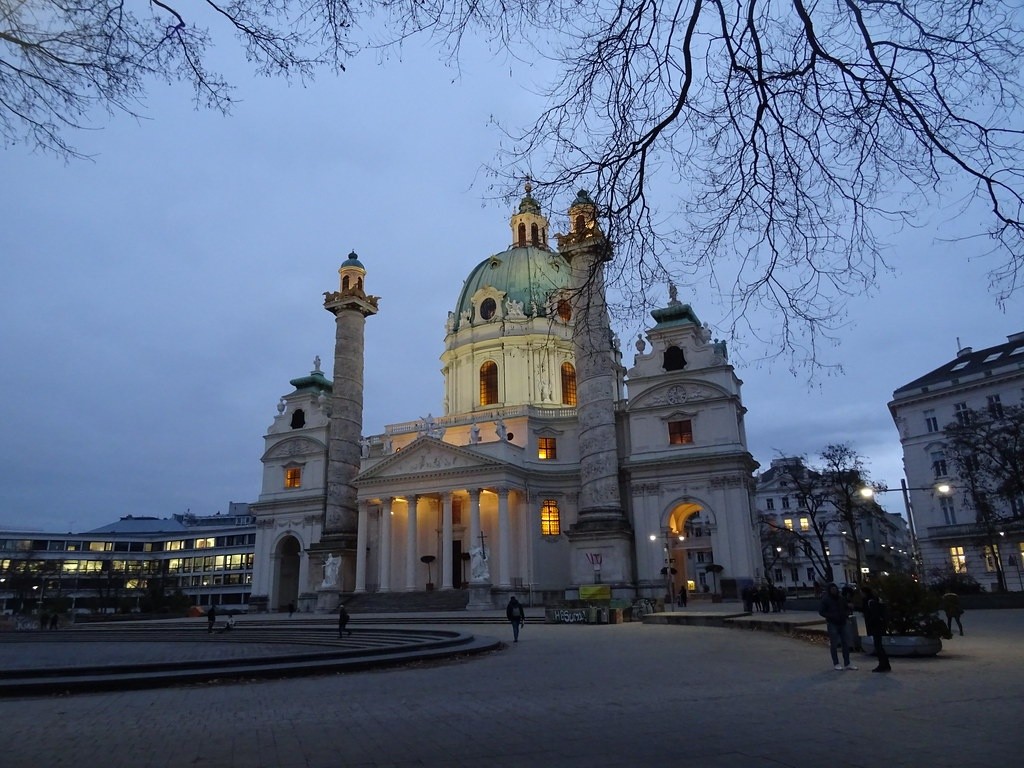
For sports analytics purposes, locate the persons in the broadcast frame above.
[470,541,490,579]
[942,586,964,637]
[742,585,786,613]
[287,599,295,618]
[506,596,525,643]
[338,605,352,638]
[40,613,49,630]
[468,423,481,443]
[860,585,892,673]
[49,614,59,630]
[361,436,370,458]
[384,434,393,455]
[414,412,446,438]
[207,604,216,634]
[224,614,235,630]
[322,553,347,588]
[679,586,687,607]
[819,583,859,671]
[494,417,508,440]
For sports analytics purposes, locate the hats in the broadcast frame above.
[829,584,837,588]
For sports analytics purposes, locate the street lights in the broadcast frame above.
[861,479,955,583]
[777,545,798,600]
[650,532,685,612]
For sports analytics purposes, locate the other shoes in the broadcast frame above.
[872,664,891,673]
[513,640,518,642]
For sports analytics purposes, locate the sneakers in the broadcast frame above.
[846,664,858,670]
[834,664,842,670]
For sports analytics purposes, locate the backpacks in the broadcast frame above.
[869,599,886,617]
[512,607,520,617]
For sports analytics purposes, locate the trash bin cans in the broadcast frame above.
[601,605,609,624]
[587,605,597,625]
[610,608,623,624]
